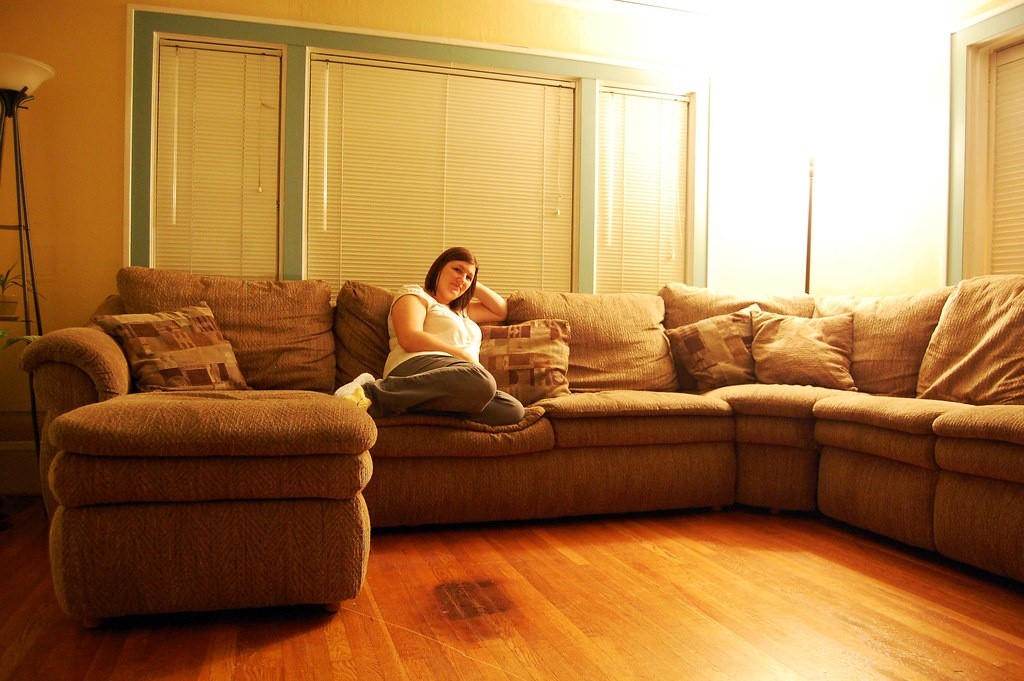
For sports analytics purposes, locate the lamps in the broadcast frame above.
[0,54,54,519]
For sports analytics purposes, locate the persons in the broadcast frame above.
[333,246,525,425]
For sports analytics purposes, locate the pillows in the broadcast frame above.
[96,264,1023,407]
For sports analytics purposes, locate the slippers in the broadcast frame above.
[335,373,382,412]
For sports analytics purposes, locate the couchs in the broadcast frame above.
[17,268,1024,632]
